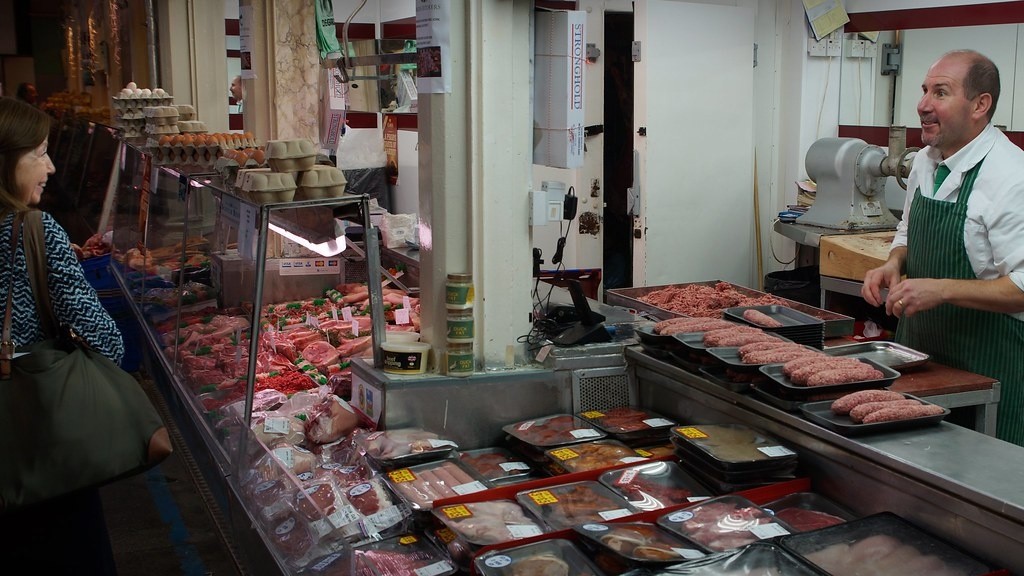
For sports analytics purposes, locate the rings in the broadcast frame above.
[897,299,905,307]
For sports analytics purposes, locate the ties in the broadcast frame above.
[933,164,951,196]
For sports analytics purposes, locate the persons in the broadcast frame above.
[860,50,1024,447]
[0,95,125,575]
[229,75,244,114]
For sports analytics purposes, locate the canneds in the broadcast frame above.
[446,272,476,376]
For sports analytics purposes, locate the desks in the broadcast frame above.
[824,336,1001,442]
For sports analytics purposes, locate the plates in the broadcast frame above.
[633,304,951,435]
[307,406,1010,576]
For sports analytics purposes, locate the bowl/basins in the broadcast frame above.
[377,332,431,375]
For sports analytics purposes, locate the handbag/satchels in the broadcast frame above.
[0,211,173,507]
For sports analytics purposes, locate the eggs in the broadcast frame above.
[121,82,164,97]
[159,130,267,167]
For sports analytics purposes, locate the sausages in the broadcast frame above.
[832,388,944,424]
[653,310,818,364]
[780,356,884,387]
